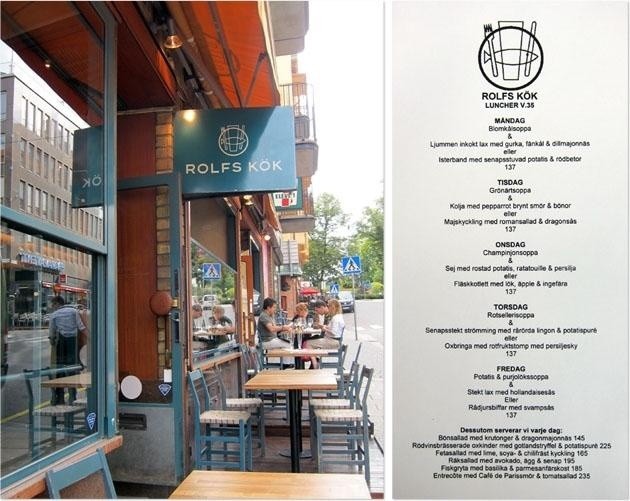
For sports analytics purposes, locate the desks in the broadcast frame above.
[167,469,374,500]
[40,372,92,445]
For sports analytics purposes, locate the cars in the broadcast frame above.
[337,289,355,313]
[190,289,265,340]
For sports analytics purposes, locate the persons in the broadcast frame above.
[191,300,240,361]
[48,297,88,406]
[258,297,345,368]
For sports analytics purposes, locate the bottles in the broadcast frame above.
[201,318,205,328]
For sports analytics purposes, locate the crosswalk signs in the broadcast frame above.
[329,283,340,294]
[202,261,223,280]
[340,255,363,273]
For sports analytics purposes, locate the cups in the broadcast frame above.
[288,329,294,349]
[297,329,303,350]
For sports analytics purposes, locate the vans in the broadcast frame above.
[0,263,15,389]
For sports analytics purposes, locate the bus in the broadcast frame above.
[8,278,90,330]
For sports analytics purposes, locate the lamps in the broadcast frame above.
[156,13,183,51]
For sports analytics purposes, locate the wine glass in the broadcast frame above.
[292,316,314,331]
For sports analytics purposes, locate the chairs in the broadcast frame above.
[24,367,84,457]
[187,313,376,483]
[11,310,51,328]
[48,363,87,444]
[42,448,119,500]
